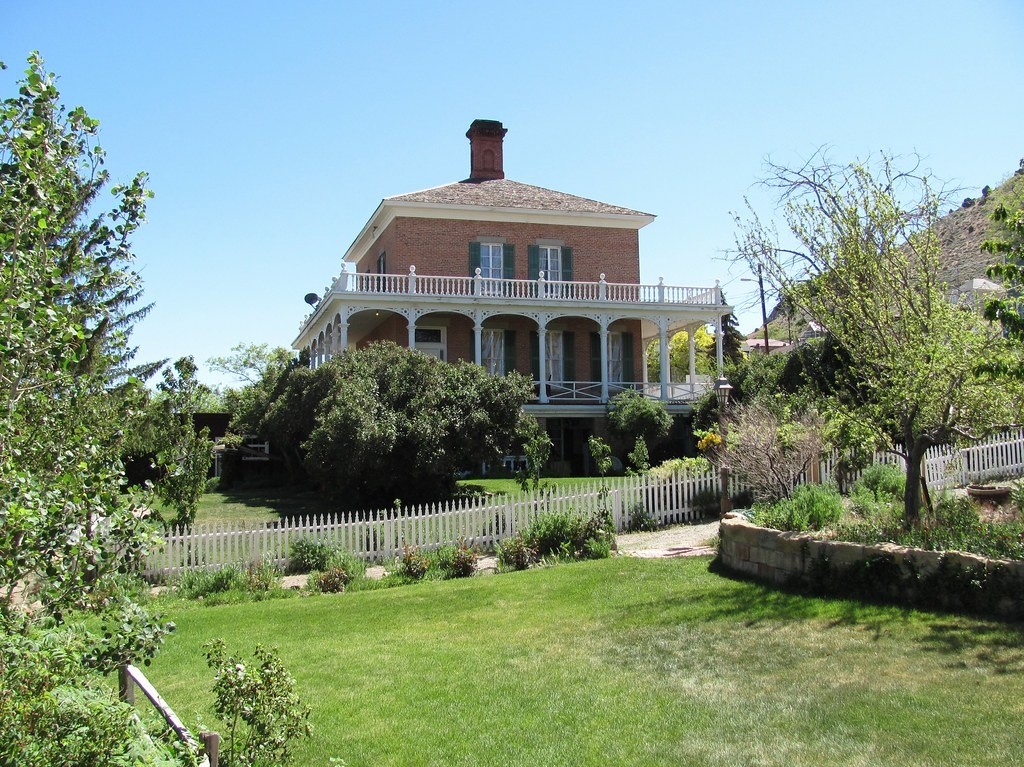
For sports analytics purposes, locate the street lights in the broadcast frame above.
[741,263,770,356]
